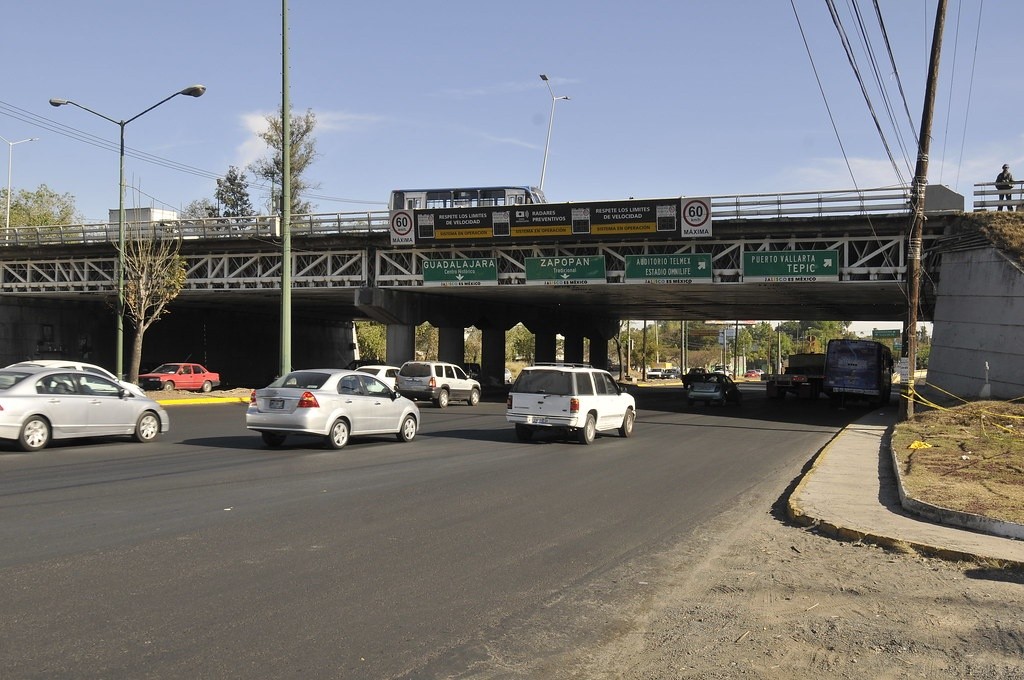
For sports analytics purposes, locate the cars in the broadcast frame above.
[505,368,512,383]
[351,365,401,394]
[138,363,220,392]
[713,368,734,379]
[0,360,146,398]
[0,366,170,451]
[756,369,764,376]
[744,370,759,378]
[661,369,681,379]
[646,368,665,379]
[687,373,741,407]
[245,369,421,449]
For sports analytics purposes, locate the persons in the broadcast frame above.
[995,164,1014,211]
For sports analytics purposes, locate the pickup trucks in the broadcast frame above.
[681,368,711,389]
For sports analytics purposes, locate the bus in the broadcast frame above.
[387,185,547,213]
[825,339,894,403]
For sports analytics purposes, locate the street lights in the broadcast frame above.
[0,135,41,246]
[539,74,570,191]
[49,84,209,388]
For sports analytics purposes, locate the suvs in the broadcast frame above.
[394,359,481,409]
[504,363,637,444]
[449,363,481,379]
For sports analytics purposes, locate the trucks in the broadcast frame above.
[759,352,827,400]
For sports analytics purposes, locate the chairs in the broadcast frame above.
[55,383,71,392]
[342,381,354,394]
[81,385,95,395]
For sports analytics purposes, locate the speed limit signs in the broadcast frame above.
[682,196,714,238]
[390,210,415,246]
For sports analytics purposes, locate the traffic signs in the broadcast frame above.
[524,255,607,285]
[624,253,714,284]
[740,249,840,282]
[420,258,498,287]
